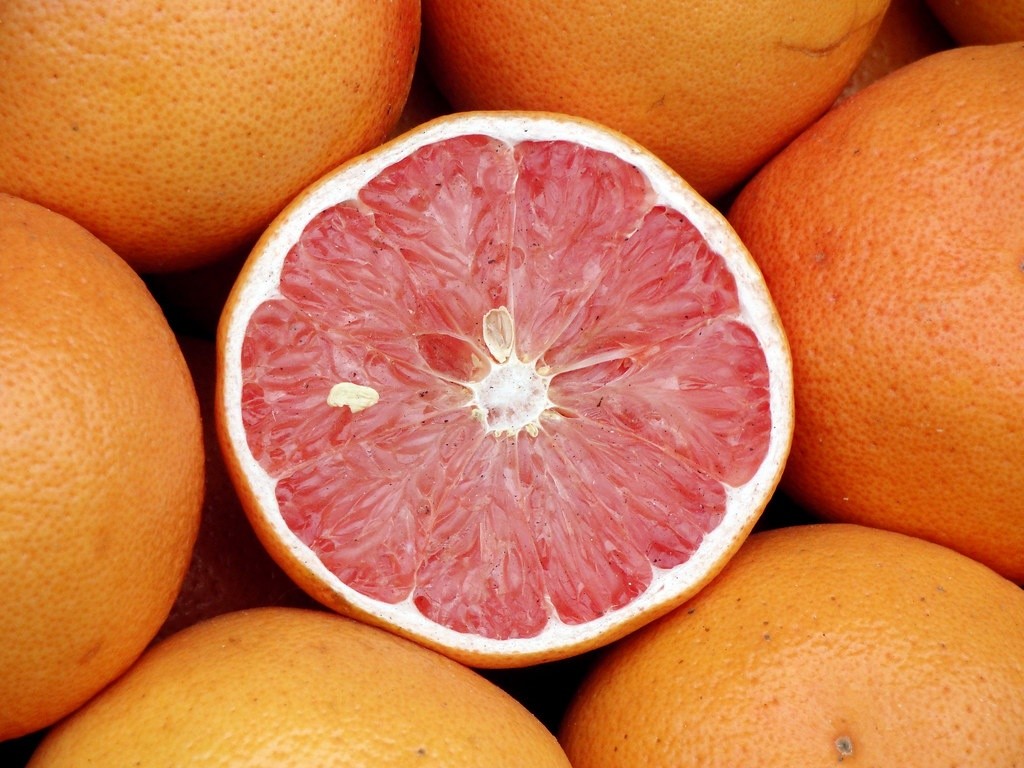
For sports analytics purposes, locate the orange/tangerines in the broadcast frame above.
[0,0,1024,768]
